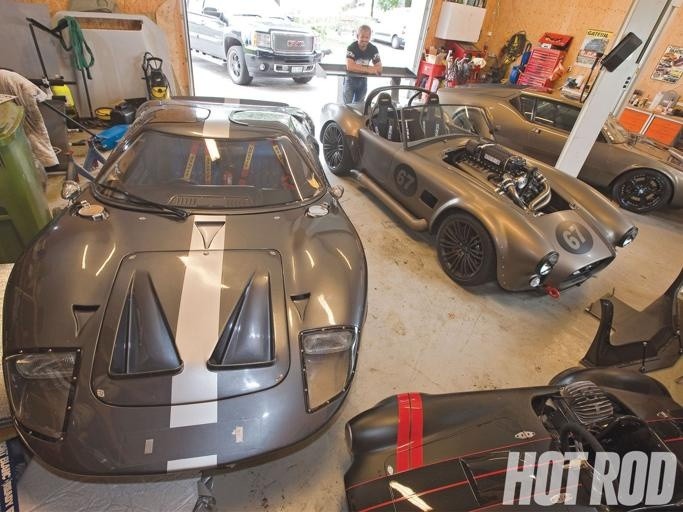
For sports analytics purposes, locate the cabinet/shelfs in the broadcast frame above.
[620,106,683,150]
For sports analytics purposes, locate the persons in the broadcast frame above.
[342,25,382,104]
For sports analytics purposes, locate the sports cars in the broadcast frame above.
[1,93,373,485]
[435,81,683,216]
[341,365,683,512]
[316,61,640,299]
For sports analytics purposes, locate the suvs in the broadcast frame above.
[185,0,323,87]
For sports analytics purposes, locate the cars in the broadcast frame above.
[362,6,411,50]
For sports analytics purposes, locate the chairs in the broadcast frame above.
[161,138,287,189]
[370,90,442,142]
